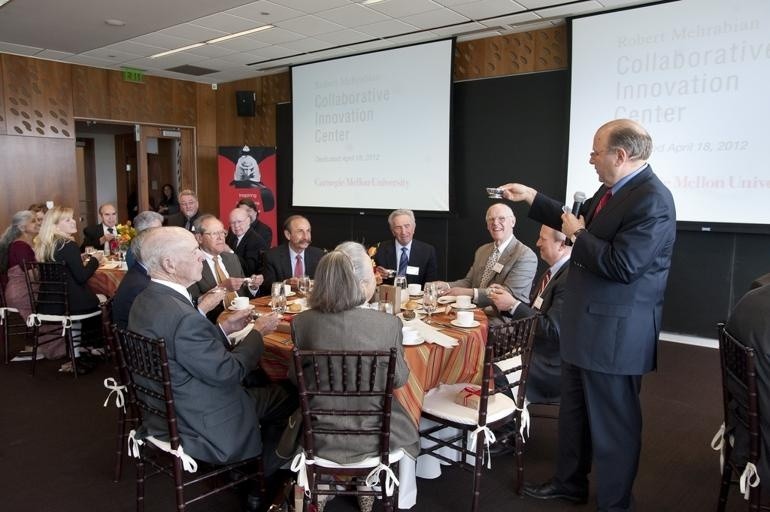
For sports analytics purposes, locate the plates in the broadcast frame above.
[395,313,419,324]
[450,318,482,329]
[286,291,297,296]
[227,303,256,312]
[410,290,424,297]
[450,301,477,310]
[437,295,456,305]
[285,306,309,314]
[400,302,423,311]
[402,332,425,346]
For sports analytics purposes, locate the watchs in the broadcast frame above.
[569,228,585,245]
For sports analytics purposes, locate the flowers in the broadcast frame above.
[103,218,140,258]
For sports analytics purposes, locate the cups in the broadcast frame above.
[378,300,393,314]
[298,274,312,293]
[271,281,283,298]
[285,286,291,293]
[231,297,250,308]
[455,294,473,306]
[408,283,422,294]
[457,310,478,324]
[272,295,288,314]
[394,275,407,291]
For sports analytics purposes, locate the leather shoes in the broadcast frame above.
[487,441,516,457]
[521,479,588,498]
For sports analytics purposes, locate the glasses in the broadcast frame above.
[204,231,228,238]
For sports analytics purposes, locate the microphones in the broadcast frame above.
[565,192,585,246]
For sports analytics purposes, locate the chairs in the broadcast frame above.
[110,320,271,510]
[290,344,405,510]
[418,309,541,512]
[521,402,557,422]
[20,260,106,381]
[1,259,34,365]
[714,319,770,512]
[103,288,143,486]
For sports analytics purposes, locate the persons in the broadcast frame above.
[750,271,770,291]
[499,120,677,512]
[426,202,538,339]
[0,202,116,366]
[219,147,276,188]
[104,187,297,510]
[723,283,769,511]
[251,209,447,510]
[480,221,574,459]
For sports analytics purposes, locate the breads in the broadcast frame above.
[402,310,416,321]
[289,303,302,312]
[406,300,418,309]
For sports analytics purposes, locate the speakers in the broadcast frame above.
[236,91,255,117]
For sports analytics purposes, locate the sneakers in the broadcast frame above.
[358,496,374,512]
[316,495,336,511]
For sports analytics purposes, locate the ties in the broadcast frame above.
[398,248,409,276]
[294,255,304,278]
[107,228,114,234]
[479,248,499,288]
[212,256,238,308]
[536,271,551,298]
[590,189,612,223]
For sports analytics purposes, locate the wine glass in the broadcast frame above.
[425,283,439,324]
[248,310,256,323]
[85,247,95,261]
[387,270,394,278]
[246,277,255,286]
[486,187,505,200]
